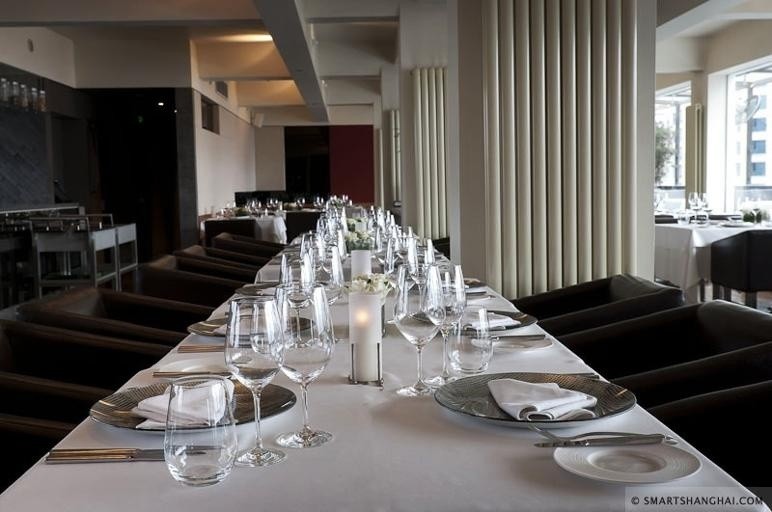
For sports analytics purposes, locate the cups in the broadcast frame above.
[444,306,492,372]
[163,376,240,488]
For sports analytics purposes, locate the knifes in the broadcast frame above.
[45,443,217,467]
[151,368,230,377]
[177,344,229,354]
[533,437,671,448]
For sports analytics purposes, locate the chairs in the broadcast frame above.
[1,186,772,511]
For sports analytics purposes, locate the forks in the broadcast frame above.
[527,420,677,440]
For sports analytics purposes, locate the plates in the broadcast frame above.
[187,315,313,339]
[472,336,553,354]
[552,433,702,485]
[88,380,297,435]
[485,309,535,332]
[433,373,638,429]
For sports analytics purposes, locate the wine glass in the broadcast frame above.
[269,280,334,449]
[279,203,434,326]
[428,263,469,386]
[738,196,765,226]
[689,193,712,229]
[393,264,445,394]
[221,296,290,469]
[216,194,348,215]
